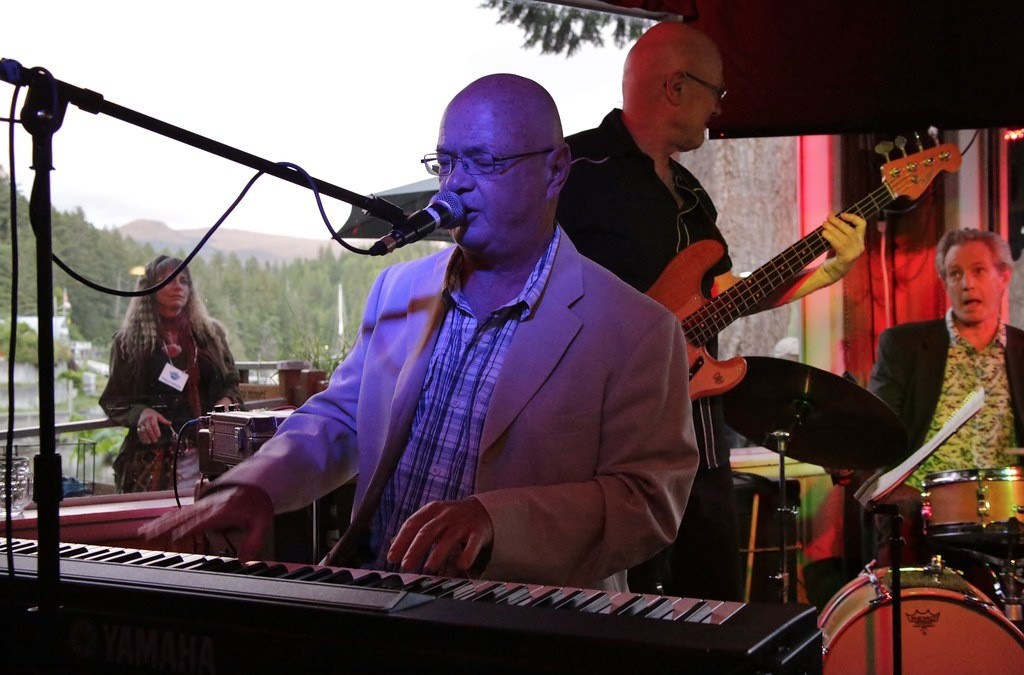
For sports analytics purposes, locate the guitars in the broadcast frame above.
[639,122,964,405]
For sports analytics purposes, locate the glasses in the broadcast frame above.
[421,149,553,177]
[663,70,728,104]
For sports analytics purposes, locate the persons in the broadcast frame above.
[136,73,700,595]
[98,256,248,493]
[551,21,869,602]
[841,229,1023,612]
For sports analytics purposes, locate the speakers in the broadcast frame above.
[730,468,802,605]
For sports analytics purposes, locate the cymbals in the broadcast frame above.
[720,354,910,471]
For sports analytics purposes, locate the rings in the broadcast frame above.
[137,428,140,434]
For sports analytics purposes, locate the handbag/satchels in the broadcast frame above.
[118,424,188,495]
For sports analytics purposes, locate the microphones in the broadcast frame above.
[370,191,465,257]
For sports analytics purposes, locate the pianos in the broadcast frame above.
[0,531,827,675]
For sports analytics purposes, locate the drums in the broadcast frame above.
[922,466,1024,544]
[816,558,1024,675]
